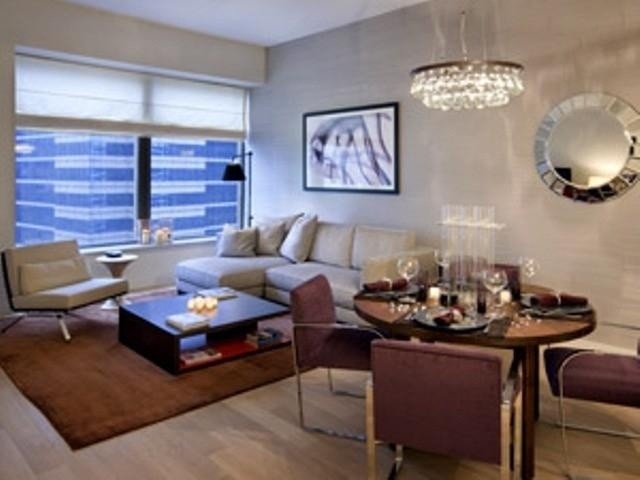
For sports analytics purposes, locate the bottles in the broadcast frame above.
[132,217,173,247]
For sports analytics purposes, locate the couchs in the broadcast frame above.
[176,220,438,327]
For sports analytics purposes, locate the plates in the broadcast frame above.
[520,297,591,318]
[411,306,488,331]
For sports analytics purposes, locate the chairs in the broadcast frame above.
[0,240,130,340]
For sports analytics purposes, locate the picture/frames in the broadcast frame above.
[303,101,400,195]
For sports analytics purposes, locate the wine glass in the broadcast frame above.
[382,250,541,317]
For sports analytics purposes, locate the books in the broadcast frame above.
[245,327,284,348]
[196,286,237,299]
[179,348,222,365]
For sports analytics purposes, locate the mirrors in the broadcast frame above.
[532,91,640,203]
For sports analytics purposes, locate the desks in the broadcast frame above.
[95,254,138,310]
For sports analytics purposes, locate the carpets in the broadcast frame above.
[0,289,320,451]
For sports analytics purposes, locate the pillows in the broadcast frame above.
[20,256,92,296]
[216,213,416,270]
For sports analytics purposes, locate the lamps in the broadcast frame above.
[223,151,253,227]
[409,0,526,110]
